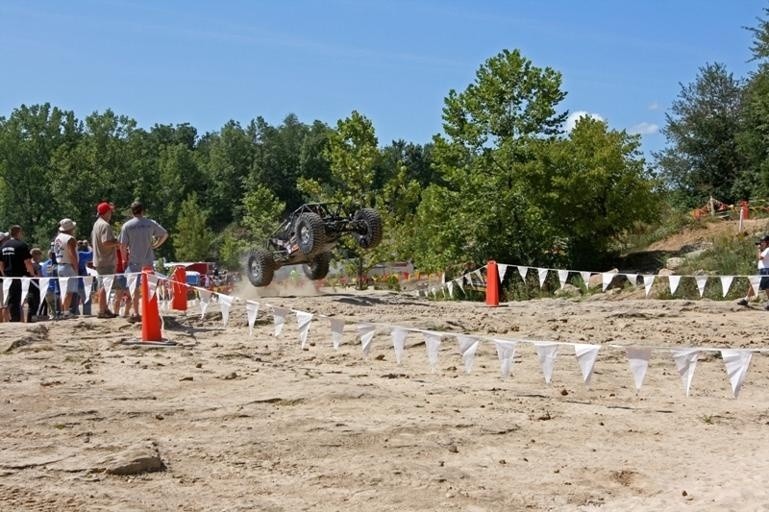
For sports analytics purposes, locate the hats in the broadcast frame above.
[58,218,77,232]
[97,203,115,216]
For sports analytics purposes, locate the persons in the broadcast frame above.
[119,201,169,320]
[90,201,120,319]
[1,224,131,323]
[737,235,769,312]
[52,218,81,319]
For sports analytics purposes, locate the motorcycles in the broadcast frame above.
[245,202,382,287]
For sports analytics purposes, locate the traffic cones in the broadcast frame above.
[475,261,508,308]
[170,266,186,311]
[123,268,176,346]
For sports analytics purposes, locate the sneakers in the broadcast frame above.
[59,311,79,320]
[126,313,141,322]
[97,310,116,318]
